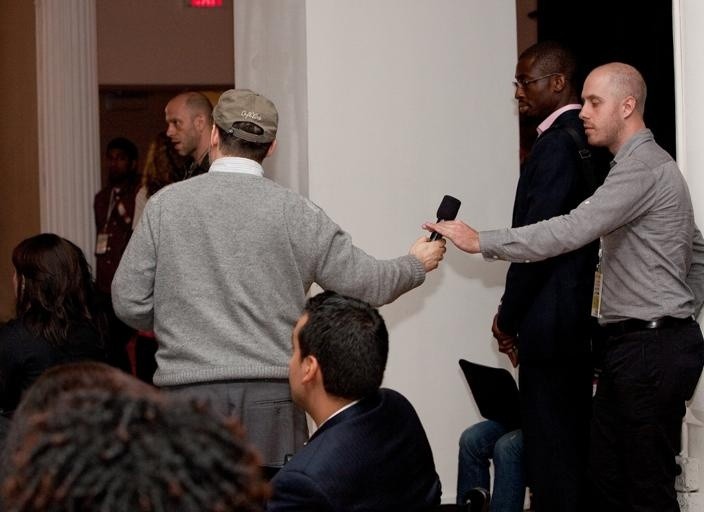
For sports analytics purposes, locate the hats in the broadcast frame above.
[212,89,279,143]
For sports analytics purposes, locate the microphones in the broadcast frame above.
[428,195,461,243]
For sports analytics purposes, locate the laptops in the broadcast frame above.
[459,359,522,426]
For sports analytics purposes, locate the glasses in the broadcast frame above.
[513,73,558,89]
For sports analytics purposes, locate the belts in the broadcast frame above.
[622,316,692,329]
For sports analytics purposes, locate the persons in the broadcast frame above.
[421,58,704,512]
[0,231,135,426]
[88,136,138,283]
[112,85,447,468]
[162,90,216,179]
[0,355,272,510]
[267,287,445,510]
[131,130,188,232]
[485,34,615,511]
[448,410,537,511]
